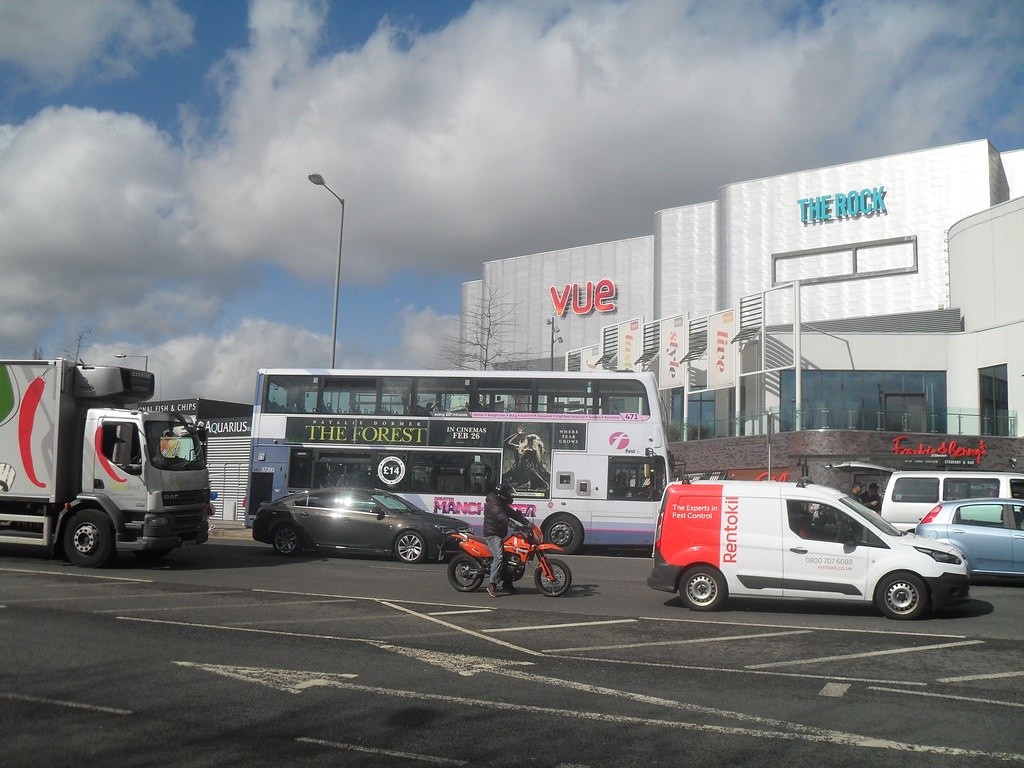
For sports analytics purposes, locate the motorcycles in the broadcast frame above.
[441,512,574,599]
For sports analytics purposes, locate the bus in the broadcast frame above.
[242,367,689,547]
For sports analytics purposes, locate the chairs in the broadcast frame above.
[294,398,397,415]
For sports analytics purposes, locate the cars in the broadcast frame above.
[916,498,1024,578]
[252,486,475,564]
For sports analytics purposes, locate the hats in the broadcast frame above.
[852,482,866,487]
[869,483,881,489]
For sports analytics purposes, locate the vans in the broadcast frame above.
[880,471,1023,538]
[650,479,973,621]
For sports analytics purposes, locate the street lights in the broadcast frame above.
[546,316,563,368]
[309,172,345,406]
[115,353,149,371]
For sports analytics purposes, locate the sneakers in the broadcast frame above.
[486,584,499,598]
[501,582,518,593]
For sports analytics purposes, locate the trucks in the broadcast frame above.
[0,358,217,567]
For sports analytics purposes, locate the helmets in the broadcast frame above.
[493,483,517,505]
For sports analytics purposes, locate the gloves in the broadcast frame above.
[510,521,521,532]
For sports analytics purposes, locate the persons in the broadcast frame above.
[208,518,215,530]
[482,485,531,597]
[803,482,882,545]
[290,402,627,498]
[502,424,550,492]
